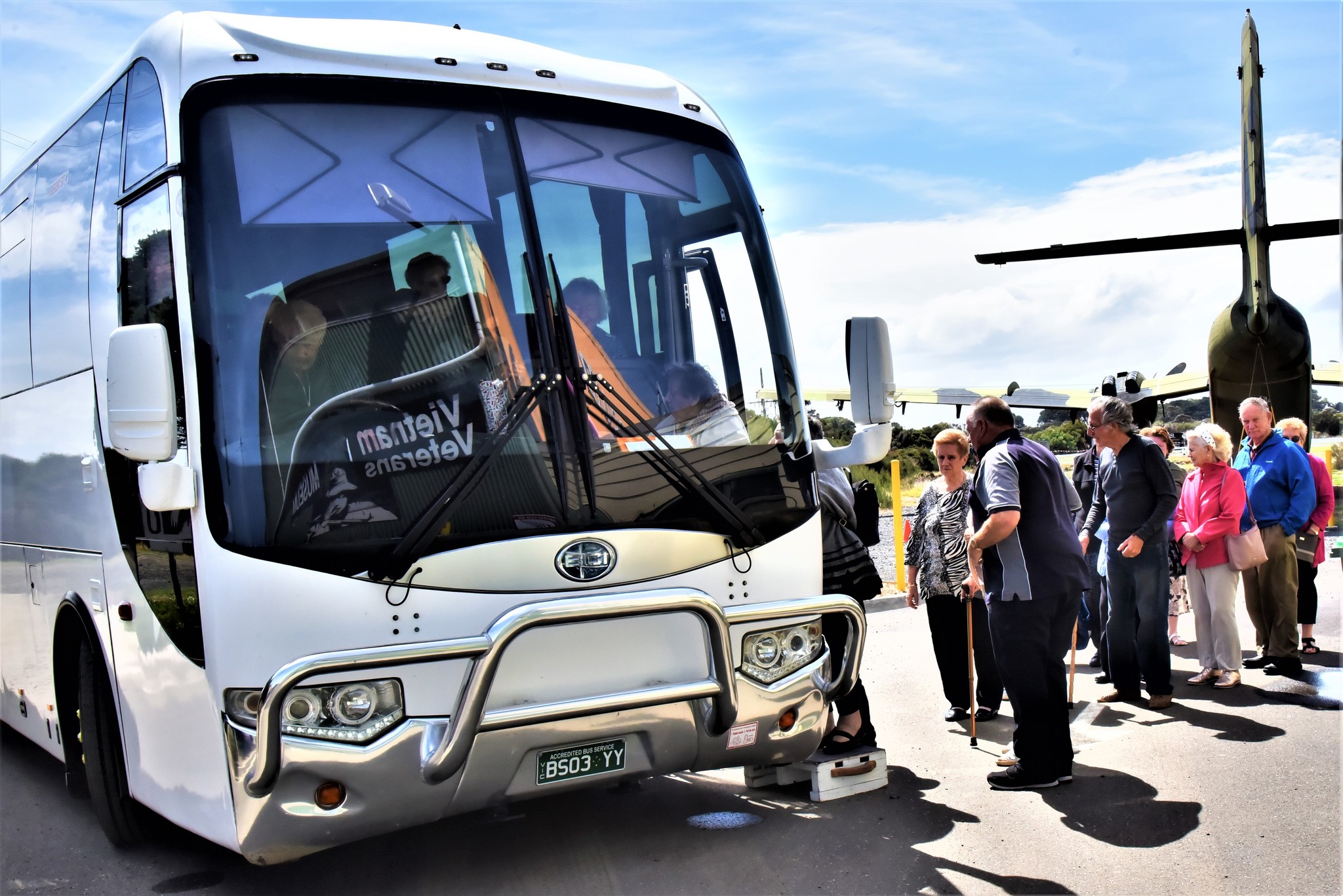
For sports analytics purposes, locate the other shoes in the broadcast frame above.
[1150,693,1173,707]
[975,708,997,721]
[821,726,836,749]
[1055,761,1073,780]
[987,762,1059,789]
[1090,650,1098,667]
[823,722,865,754]
[1263,656,1302,674]
[1243,654,1270,667]
[945,706,969,720]
[1097,691,1122,702]
[1095,669,1110,683]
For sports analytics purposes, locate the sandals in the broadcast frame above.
[998,749,1021,765]
[1169,634,1192,645]
[1302,637,1320,654]
[1002,741,1014,754]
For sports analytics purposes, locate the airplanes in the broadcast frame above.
[754,360,1343,426]
[971,8,1343,446]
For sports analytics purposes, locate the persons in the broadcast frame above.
[904,429,1004,720]
[1138,426,1188,645]
[387,254,489,330]
[1072,439,1146,682]
[769,417,878,758]
[1274,417,1335,653]
[1172,423,1247,688]
[963,395,1092,789]
[258,300,336,429]
[559,277,633,362]
[1077,395,1179,709]
[1234,397,1316,677]
[650,361,750,448]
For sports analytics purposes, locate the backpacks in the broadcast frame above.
[822,466,881,546]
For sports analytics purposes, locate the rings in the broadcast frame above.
[1201,545,1203,547]
[1131,555,1134,557]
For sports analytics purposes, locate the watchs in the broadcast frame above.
[971,537,982,551]
[1309,527,1319,535]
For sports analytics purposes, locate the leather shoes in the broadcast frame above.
[1214,667,1241,688]
[1188,664,1222,685]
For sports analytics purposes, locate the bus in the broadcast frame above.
[0,13,899,873]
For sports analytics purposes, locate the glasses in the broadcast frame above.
[1282,436,1303,443]
[421,275,451,289]
[1083,418,1117,430]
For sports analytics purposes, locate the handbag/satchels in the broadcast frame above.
[1065,593,1090,650]
[1167,540,1189,577]
[1295,531,1321,563]
[1221,468,1269,572]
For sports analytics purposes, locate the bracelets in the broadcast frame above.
[969,573,972,576]
[907,582,917,588]
